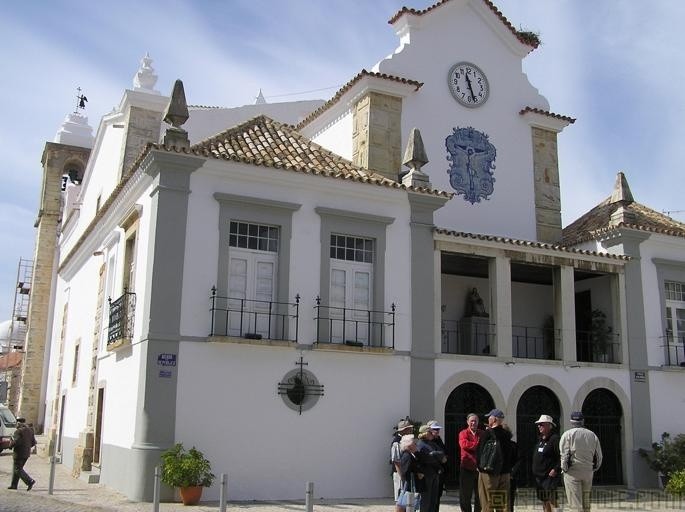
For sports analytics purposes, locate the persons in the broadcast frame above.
[8,417,36,491]
[459,409,523,512]
[391,420,447,512]
[532,415,562,512]
[559,412,602,512]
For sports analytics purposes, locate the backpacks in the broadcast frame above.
[479,426,504,473]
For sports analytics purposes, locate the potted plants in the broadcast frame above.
[158,441,217,505]
[589,305,613,363]
[633,432,685,488]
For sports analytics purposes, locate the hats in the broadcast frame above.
[535,415,557,427]
[396,420,443,433]
[571,412,584,420]
[484,409,504,418]
[16,417,25,423]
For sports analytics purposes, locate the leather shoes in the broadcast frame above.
[27,479,35,491]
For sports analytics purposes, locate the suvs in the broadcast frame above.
[0,404,19,453]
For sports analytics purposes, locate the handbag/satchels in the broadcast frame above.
[397,473,421,510]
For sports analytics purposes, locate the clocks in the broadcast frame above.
[448,62,489,107]
